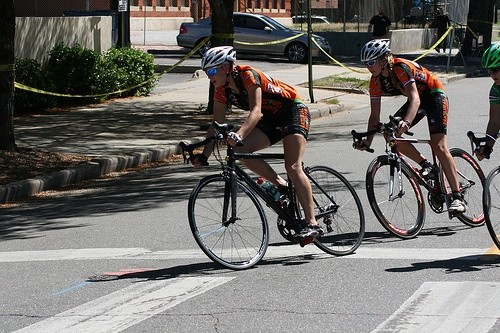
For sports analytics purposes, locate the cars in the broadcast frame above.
[175,11,332,64]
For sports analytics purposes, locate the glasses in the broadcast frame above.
[363,58,381,67]
[206,65,223,76]
[486,67,500,72]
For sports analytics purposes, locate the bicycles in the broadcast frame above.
[174,122,366,272]
[349,114,493,240]
[464,129,500,250]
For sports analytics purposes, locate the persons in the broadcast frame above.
[189,46,326,242]
[368,7,391,39]
[474,44,500,162]
[433,8,451,52]
[353,38,468,213]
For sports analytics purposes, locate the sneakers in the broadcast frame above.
[293,223,325,240]
[418,165,436,179]
[448,192,467,212]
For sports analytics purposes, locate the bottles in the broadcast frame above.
[412,167,424,178]
[257,176,281,203]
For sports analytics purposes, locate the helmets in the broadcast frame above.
[201,46,237,70]
[481,44,500,69]
[361,39,391,64]
